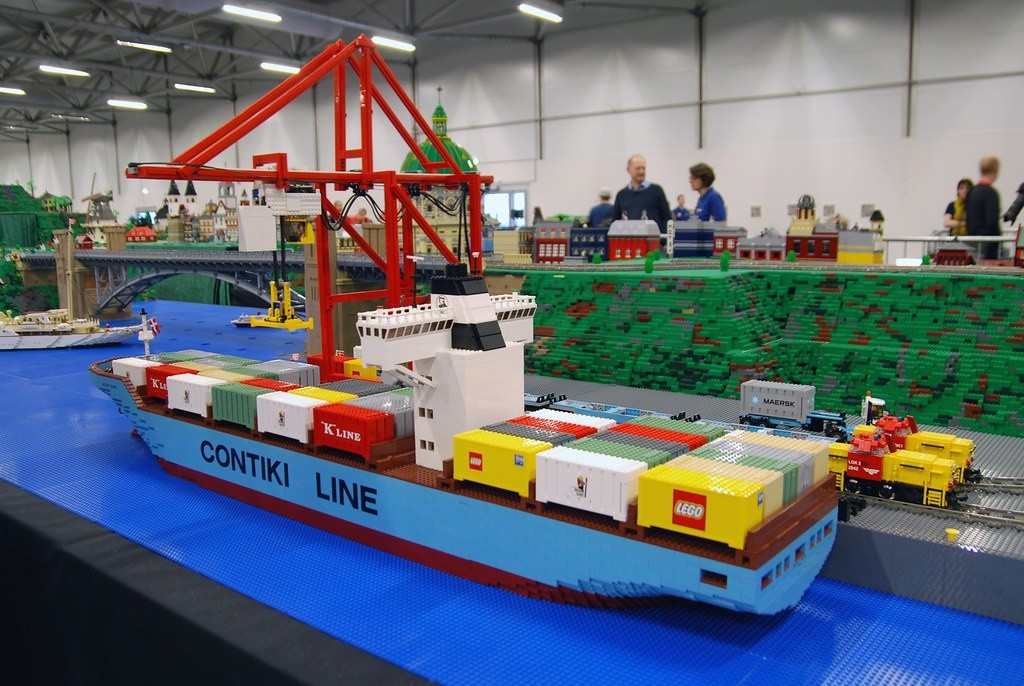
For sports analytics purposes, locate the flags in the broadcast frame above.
[69,219,76,224]
[336,350,343,356]
[149,318,160,337]
[4,237,59,261]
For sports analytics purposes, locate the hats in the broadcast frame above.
[600,187,612,197]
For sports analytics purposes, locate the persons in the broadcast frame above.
[532,206,543,225]
[334,200,342,211]
[672,191,688,221]
[965,156,1000,259]
[588,191,615,228]
[615,154,671,234]
[943,178,972,236]
[357,209,371,222]
[688,163,727,228]
